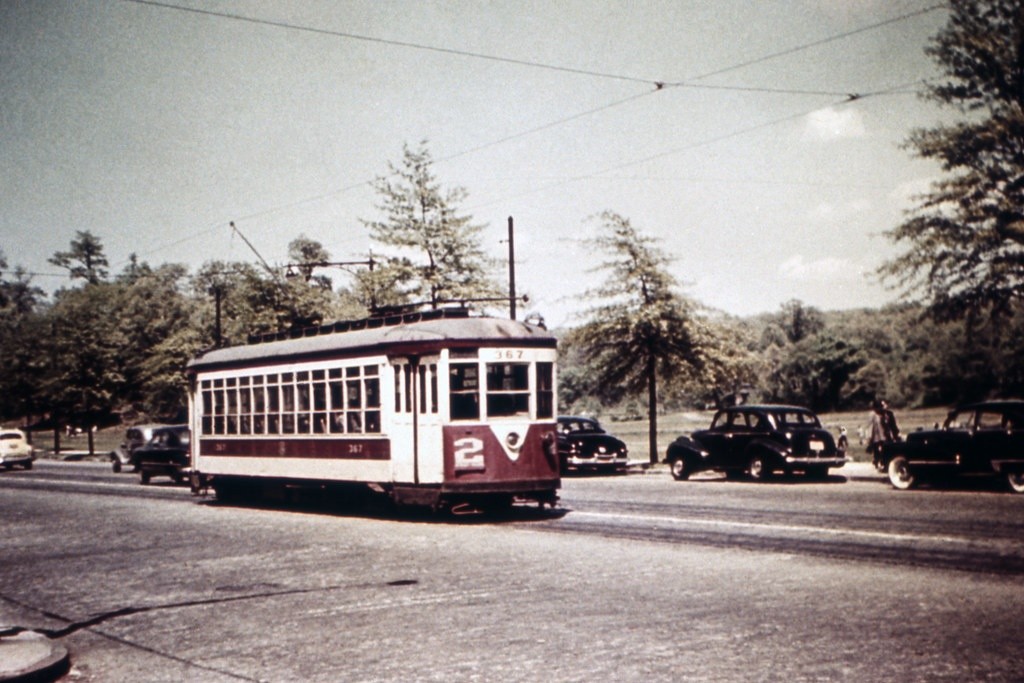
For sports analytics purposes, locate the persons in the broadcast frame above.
[837,427,849,451]
[215,400,380,434]
[860,400,901,467]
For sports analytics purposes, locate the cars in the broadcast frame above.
[661,406,849,483]
[557,415,628,476]
[129,424,192,483]
[111,424,156,472]
[864,399,1024,494]
[0,426,34,470]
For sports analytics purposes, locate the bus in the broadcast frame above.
[183,222,561,520]
